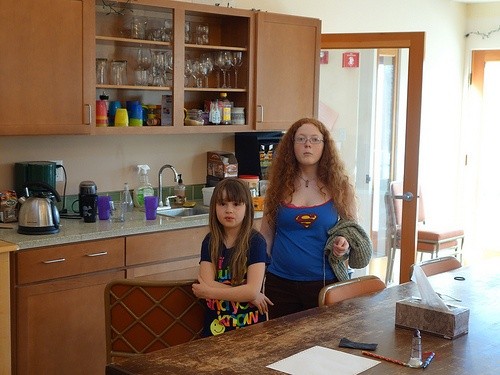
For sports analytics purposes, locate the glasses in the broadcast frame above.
[293,135,325,144]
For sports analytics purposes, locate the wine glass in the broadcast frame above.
[184,51,244,89]
[132,49,174,87]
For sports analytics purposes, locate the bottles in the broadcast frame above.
[217,93,232,125]
[407,329,423,368]
[230,107,246,126]
[239,174,260,199]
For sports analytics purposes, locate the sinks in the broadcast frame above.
[156,205,210,219]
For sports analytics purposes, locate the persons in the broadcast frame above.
[258,118,356,320]
[191,177,274,338]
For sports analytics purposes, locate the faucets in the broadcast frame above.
[158,164,178,207]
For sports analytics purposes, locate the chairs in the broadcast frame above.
[416,256,462,277]
[319,182,464,306]
[102,278,206,367]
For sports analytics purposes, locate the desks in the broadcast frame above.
[105,266,500,375]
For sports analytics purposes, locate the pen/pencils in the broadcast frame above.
[423,352,435,369]
[362,350,408,367]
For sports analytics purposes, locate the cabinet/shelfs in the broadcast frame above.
[0,0,97,137]
[10,216,262,375]
[254,10,322,131]
[94,0,255,134]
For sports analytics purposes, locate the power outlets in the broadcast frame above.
[53,160,64,181]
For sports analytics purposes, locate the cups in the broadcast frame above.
[78,181,98,218]
[96,99,148,128]
[97,195,112,220]
[95,57,129,85]
[80,194,98,223]
[184,21,210,45]
[144,196,158,220]
[259,180,270,196]
[128,15,174,41]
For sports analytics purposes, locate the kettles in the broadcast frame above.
[17,182,61,235]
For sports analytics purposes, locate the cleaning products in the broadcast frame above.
[173,173,186,206]
[133,164,155,209]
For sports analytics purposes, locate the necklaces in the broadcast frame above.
[299,175,318,187]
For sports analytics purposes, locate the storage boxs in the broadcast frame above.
[202,187,216,207]
[207,151,238,178]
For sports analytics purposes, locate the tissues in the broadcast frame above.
[395,265,470,341]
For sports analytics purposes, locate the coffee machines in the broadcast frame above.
[15,160,56,218]
